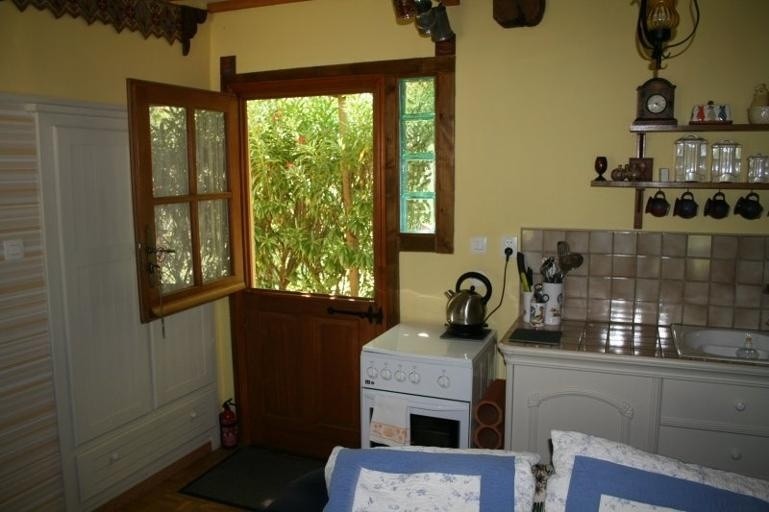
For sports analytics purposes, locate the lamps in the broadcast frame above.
[391,0,456,41]
[631,0,700,69]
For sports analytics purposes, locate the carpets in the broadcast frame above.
[178,447,328,512]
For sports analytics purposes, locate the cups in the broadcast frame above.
[521,281,563,328]
[644,190,763,220]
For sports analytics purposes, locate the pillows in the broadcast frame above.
[540,426,769,512]
[324,443,538,512]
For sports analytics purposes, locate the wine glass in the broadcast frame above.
[593,156,611,180]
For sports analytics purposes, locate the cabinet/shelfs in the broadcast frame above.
[503,352,662,469]
[39,115,226,512]
[589,124,769,189]
[652,360,769,484]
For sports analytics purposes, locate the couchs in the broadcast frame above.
[262,458,769,512]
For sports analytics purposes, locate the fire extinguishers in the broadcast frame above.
[220,398,240,450]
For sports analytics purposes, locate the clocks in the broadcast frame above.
[635,76,677,125]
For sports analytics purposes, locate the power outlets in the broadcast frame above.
[500,236,517,258]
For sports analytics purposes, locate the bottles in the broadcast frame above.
[612,164,645,181]
[748,152,768,185]
[747,84,769,124]
[676,134,709,182]
[711,139,744,184]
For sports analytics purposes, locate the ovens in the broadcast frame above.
[359,387,471,451]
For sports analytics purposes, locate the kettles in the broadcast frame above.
[444,271,492,328]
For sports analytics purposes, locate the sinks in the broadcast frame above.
[671,324,769,370]
